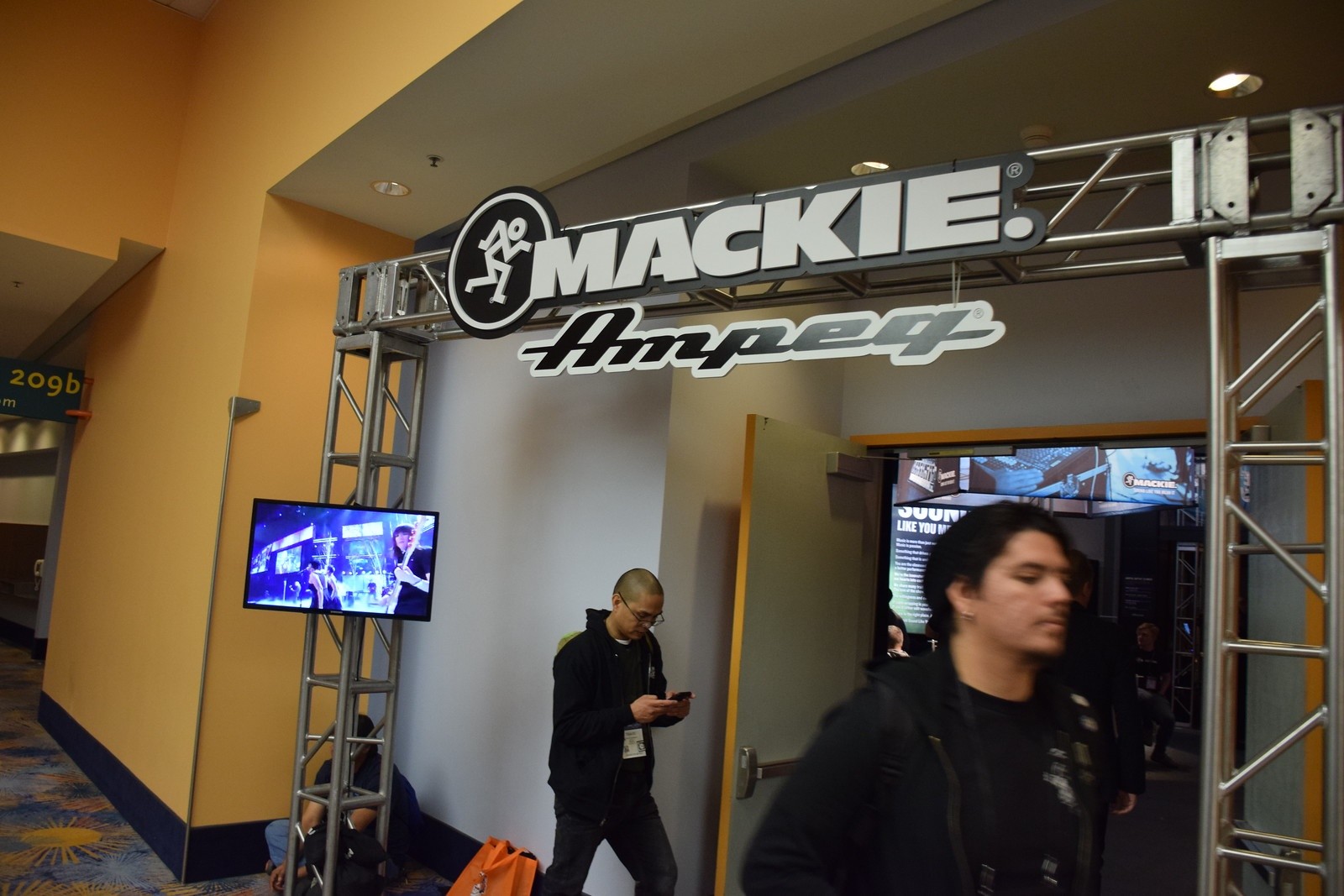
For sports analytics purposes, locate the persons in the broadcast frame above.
[734,500,1146,896]
[533,571,699,896]
[1127,617,1182,769]
[260,712,420,896]
[968,458,1044,497]
[252,524,435,620]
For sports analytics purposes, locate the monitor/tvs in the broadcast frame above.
[242,498,441,623]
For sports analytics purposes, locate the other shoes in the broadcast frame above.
[1152,750,1178,767]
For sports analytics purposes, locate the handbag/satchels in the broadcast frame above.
[445,835,539,896]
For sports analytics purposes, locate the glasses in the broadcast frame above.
[618,591,665,628]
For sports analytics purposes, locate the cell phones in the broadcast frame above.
[668,691,692,700]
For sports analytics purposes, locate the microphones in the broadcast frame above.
[297,566,309,574]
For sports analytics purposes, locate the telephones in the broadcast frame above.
[34,559,43,576]
